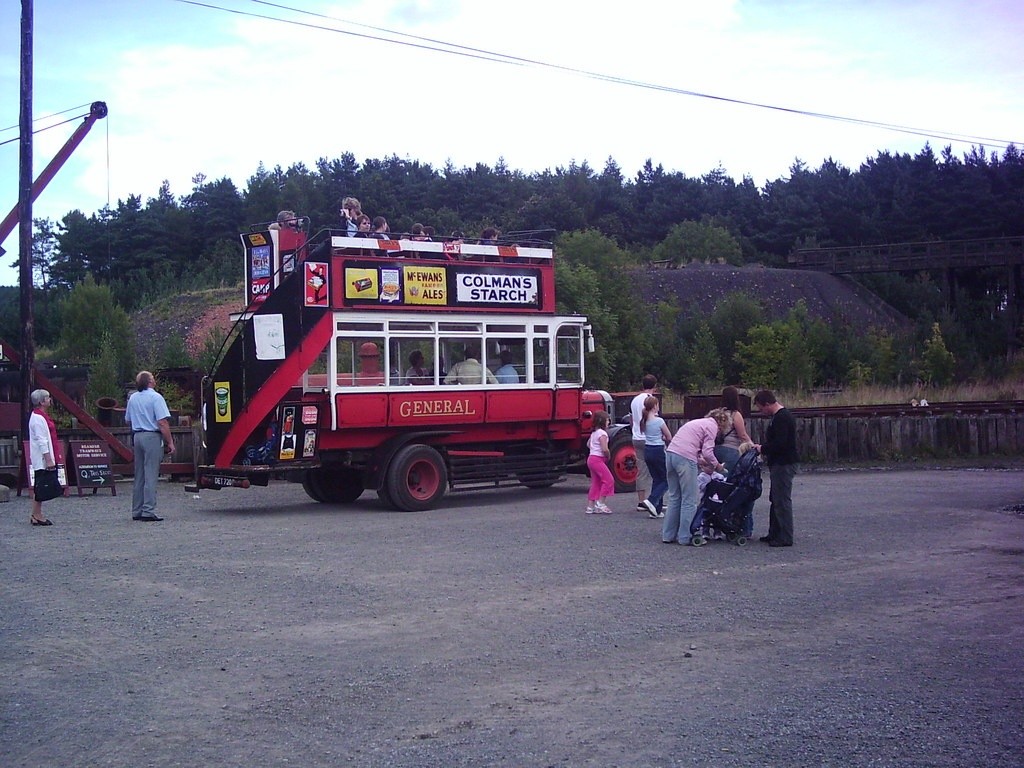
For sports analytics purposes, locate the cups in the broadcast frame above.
[215,387,229,416]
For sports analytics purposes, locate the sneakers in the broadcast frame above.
[642,499,657,515]
[649,512,664,519]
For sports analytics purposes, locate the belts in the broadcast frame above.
[136,430,161,433]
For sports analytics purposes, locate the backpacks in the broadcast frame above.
[716,409,735,445]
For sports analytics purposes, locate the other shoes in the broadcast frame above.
[769,540,792,547]
[701,535,710,541]
[661,504,668,509]
[715,535,722,539]
[637,502,648,511]
[759,535,774,541]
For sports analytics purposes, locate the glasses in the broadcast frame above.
[361,222,370,225]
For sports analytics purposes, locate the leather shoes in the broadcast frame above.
[142,515,164,522]
[133,515,142,520]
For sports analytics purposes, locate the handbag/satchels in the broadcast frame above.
[33,466,64,502]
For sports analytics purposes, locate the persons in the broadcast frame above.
[584,410,615,514]
[28,389,61,525]
[405,346,519,385]
[629,374,800,546]
[269,197,524,263]
[125,371,176,521]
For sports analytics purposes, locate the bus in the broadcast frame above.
[184,216,638,511]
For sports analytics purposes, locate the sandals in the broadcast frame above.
[30,515,53,526]
[595,501,612,513]
[585,507,596,514]
